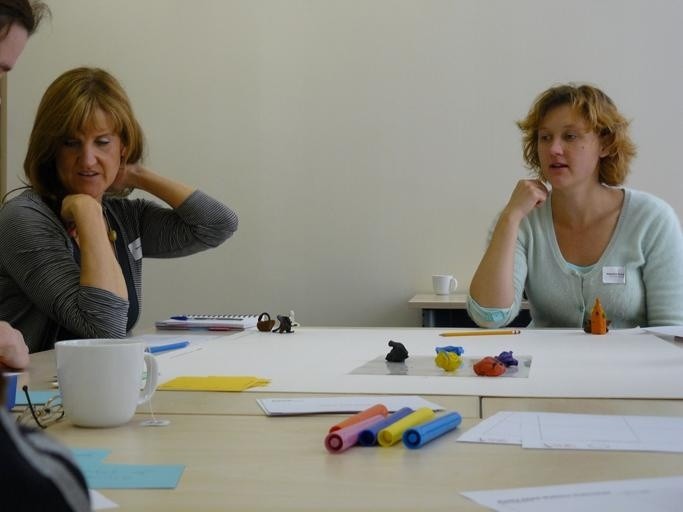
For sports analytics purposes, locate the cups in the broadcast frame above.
[432,275,458,295]
[54,338,160,430]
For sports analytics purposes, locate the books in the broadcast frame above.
[157,313,263,331]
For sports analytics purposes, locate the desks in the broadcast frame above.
[408,293,530,327]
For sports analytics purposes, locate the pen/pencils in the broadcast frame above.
[170,317,243,320]
[439,330,521,337]
[145,341,189,353]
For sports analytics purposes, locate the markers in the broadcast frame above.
[325,404,462,455]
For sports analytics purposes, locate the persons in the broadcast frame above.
[466,83,683,330]
[1,68,238,355]
[0,0,51,368]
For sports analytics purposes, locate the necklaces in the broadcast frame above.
[68,206,119,264]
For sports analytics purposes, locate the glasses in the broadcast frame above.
[18,386,65,428]
